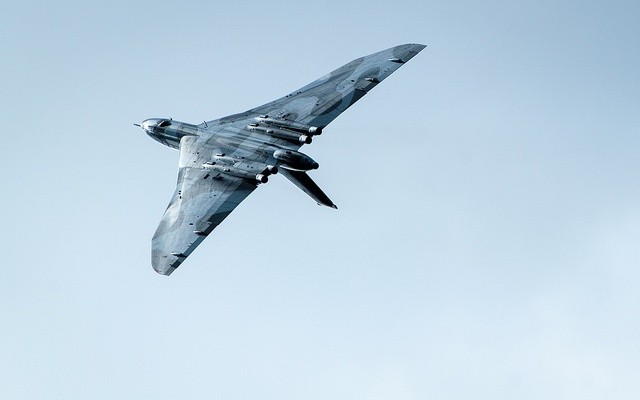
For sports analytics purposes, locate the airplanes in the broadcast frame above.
[133,44,429,276]
